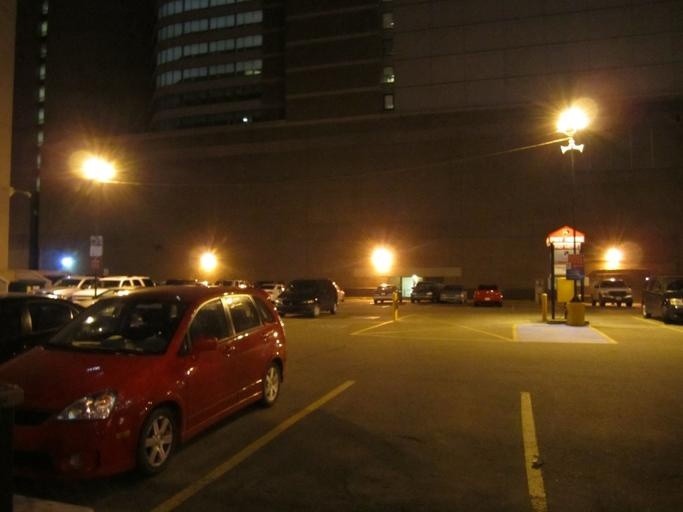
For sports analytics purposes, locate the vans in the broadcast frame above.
[642,274,683,323]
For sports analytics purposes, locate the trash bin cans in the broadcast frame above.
[566,302,585,326]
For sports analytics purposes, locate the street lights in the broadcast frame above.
[555,109,590,304]
[66,146,126,301]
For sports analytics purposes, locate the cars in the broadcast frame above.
[369,280,505,308]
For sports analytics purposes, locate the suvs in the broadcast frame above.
[591,277,634,308]
